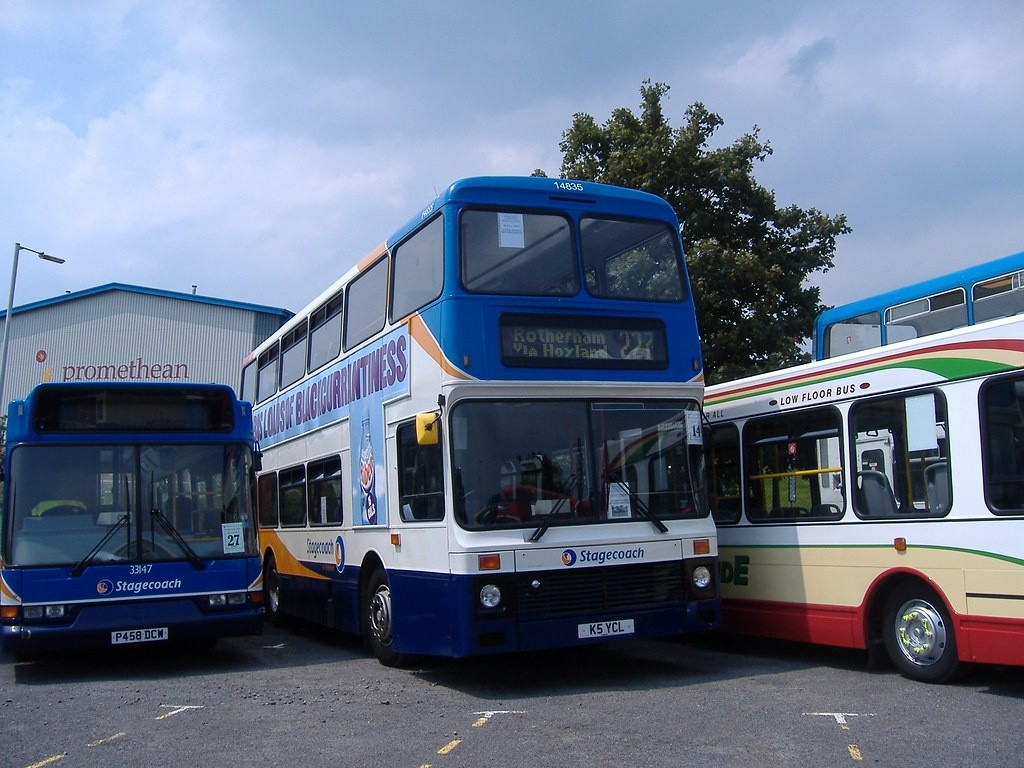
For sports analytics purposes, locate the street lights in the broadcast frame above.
[0,241,66,412]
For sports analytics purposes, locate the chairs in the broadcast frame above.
[718,495,841,519]
[856,470,898,516]
[168,497,193,531]
[924,463,951,513]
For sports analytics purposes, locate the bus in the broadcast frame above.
[597,313,1024,685]
[234,173,728,671]
[0,380,269,646]
[810,249,1024,511]
